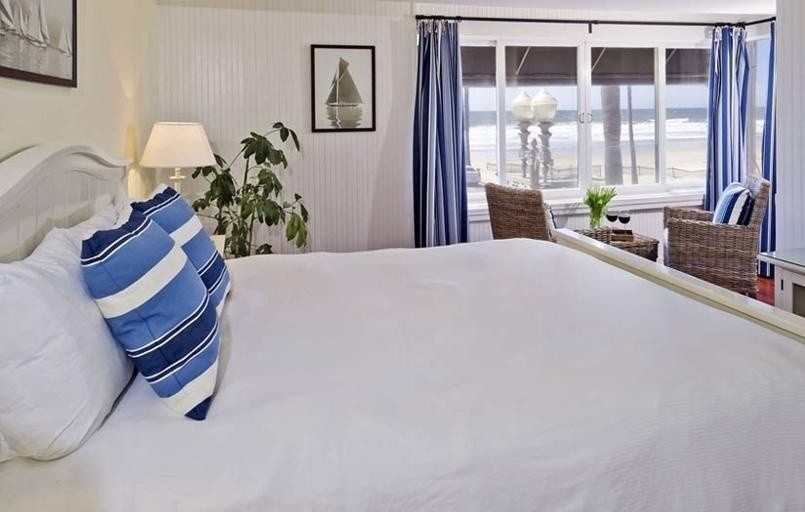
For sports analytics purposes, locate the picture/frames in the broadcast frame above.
[0,1,80,86]
[309,43,378,133]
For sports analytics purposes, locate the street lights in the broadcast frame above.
[512,85,559,193]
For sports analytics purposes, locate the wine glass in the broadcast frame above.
[606,208,617,235]
[619,209,630,234]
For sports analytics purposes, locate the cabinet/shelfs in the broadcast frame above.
[755,252,805,321]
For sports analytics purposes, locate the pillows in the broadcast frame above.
[1,225,135,461]
[58,192,118,254]
[129,183,232,319]
[712,181,754,225]
[82,205,221,421]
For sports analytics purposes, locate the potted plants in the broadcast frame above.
[585,185,617,229]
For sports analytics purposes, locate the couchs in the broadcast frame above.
[485,183,611,250]
[663,176,770,300]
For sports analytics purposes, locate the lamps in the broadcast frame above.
[138,120,215,198]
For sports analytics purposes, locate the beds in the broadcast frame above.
[1,226,805,512]
